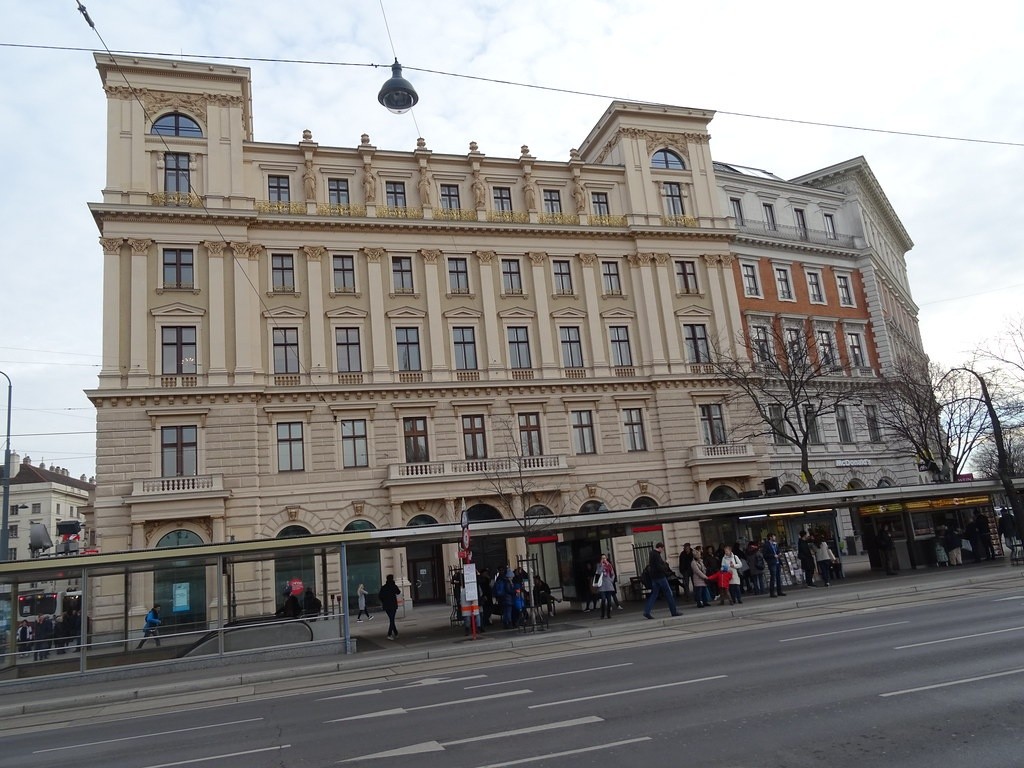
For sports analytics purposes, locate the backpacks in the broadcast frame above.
[640,565,652,590]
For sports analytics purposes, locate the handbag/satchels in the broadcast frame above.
[754,551,765,570]
[734,555,750,574]
[592,571,603,588]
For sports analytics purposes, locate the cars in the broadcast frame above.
[994,505,1015,517]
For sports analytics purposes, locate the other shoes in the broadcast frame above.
[357,620,363,623]
[643,613,655,619]
[395,632,399,639]
[617,605,623,609]
[388,635,395,640]
[777,590,786,596]
[369,616,374,620]
[672,612,683,616]
[808,583,817,588]
[584,608,591,613]
[770,591,778,598]
[696,601,743,608]
[610,607,613,611]
[549,611,554,617]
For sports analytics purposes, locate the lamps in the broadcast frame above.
[377,58,419,114]
[54,519,81,535]
[29,523,56,559]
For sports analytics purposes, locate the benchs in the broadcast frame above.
[629,576,651,601]
[525,598,555,616]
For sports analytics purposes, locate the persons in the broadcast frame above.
[878,523,897,576]
[642,542,684,619]
[678,533,766,609]
[583,553,623,620]
[16,609,90,660]
[136,605,162,650]
[356,584,374,622]
[379,575,401,640]
[451,565,563,635]
[275,589,321,623]
[798,530,837,588]
[763,532,786,598]
[935,508,1018,567]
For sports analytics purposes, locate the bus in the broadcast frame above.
[15,590,82,641]
